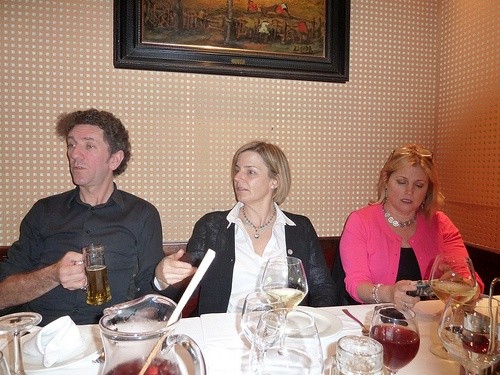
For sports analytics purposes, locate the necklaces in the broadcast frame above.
[243,205,276,239]
[382,207,417,227]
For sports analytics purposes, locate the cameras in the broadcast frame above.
[409,279,435,297]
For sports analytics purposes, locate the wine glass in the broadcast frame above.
[369,303,420,375]
[0,312,43,375]
[428,253,479,363]
[260,256,308,366]
[437,293,500,375]
[240,292,287,372]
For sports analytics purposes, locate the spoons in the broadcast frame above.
[342,309,370,337]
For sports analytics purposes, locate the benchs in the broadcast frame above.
[0,236,500,318]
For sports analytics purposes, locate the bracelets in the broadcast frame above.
[373,284,383,304]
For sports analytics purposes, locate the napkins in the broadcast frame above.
[23,315,83,368]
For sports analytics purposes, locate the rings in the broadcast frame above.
[404,304,409,308]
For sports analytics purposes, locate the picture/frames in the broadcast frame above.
[111,0,350,83]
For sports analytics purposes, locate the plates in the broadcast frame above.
[251,306,343,340]
[3,326,105,372]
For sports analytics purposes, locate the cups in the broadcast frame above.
[249,308,324,375]
[0,350,12,375]
[330,335,384,375]
[73,243,112,306]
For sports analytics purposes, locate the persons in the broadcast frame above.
[340,145,484,311]
[152,142,339,322]
[0,108,177,328]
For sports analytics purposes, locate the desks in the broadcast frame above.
[1,295,500,375]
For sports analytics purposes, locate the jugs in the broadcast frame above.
[99,293,207,375]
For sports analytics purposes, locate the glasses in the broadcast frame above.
[387,147,433,164]
